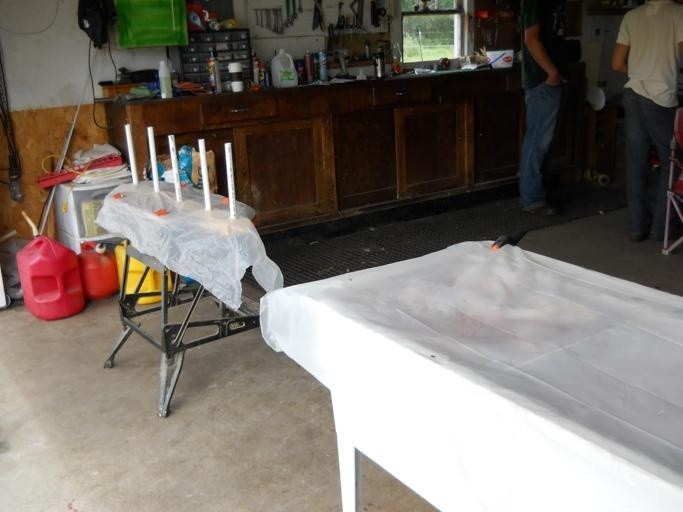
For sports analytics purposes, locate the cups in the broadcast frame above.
[227,63,242,92]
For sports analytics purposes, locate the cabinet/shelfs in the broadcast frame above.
[166,27,253,91]
[103,62,525,233]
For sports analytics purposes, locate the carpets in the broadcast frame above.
[238,189,682,314]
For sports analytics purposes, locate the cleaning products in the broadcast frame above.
[271,49,299,88]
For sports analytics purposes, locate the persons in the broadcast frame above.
[612,1,682,241]
[519,1,564,216]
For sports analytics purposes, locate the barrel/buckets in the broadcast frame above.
[115,241,173,304]
[75,241,120,298]
[486,50,513,68]
[16,235,85,322]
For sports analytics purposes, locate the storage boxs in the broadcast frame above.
[56,229,133,264]
[52,172,138,241]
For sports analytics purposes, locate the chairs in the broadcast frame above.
[662,108,683,258]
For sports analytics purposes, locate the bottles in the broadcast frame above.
[375,52,383,79]
[392,42,402,74]
[253,56,270,87]
[297,49,328,84]
[207,51,221,94]
[363,40,370,60]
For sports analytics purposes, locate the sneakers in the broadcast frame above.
[525,204,561,218]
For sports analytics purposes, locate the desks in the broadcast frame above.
[246,234,681,512]
